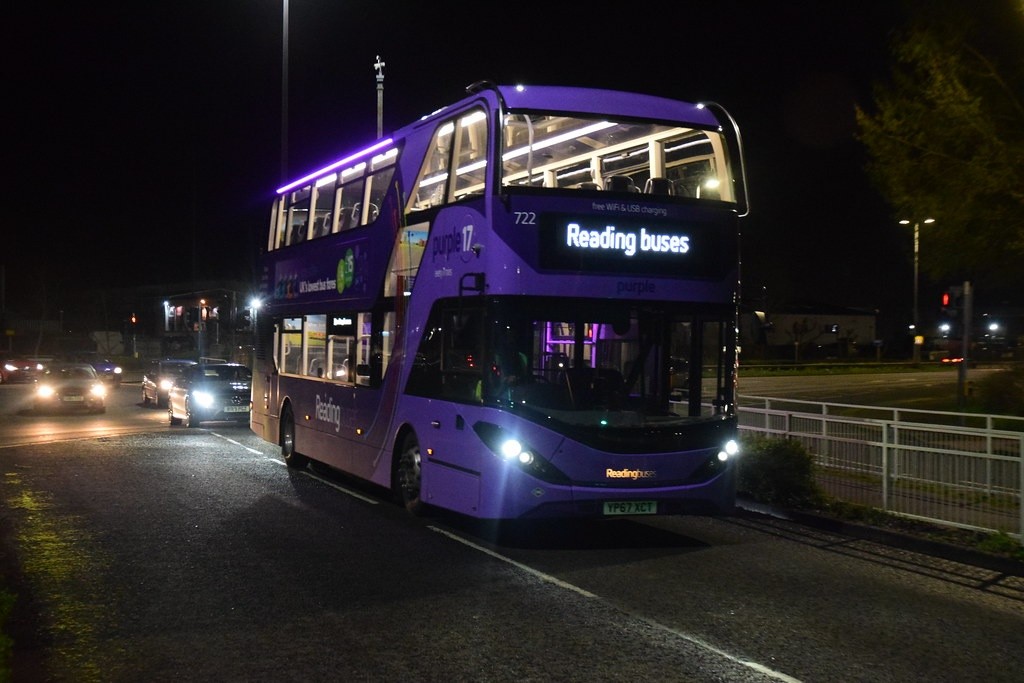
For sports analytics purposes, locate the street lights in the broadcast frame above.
[899,217,936,360]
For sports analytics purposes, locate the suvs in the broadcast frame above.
[140,359,198,406]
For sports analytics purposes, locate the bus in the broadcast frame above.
[250,80,749,523]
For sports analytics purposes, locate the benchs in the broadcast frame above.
[296,351,348,374]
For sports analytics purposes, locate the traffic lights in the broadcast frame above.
[130,316,137,324]
[941,288,957,305]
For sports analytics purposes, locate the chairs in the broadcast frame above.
[338,207,359,231]
[343,358,365,368]
[296,220,307,242]
[644,178,675,196]
[603,175,636,193]
[349,202,379,228]
[321,212,345,236]
[576,183,602,190]
[333,363,348,382]
[313,216,330,238]
[309,358,326,377]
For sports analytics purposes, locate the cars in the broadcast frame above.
[0,350,121,415]
[168,363,252,428]
